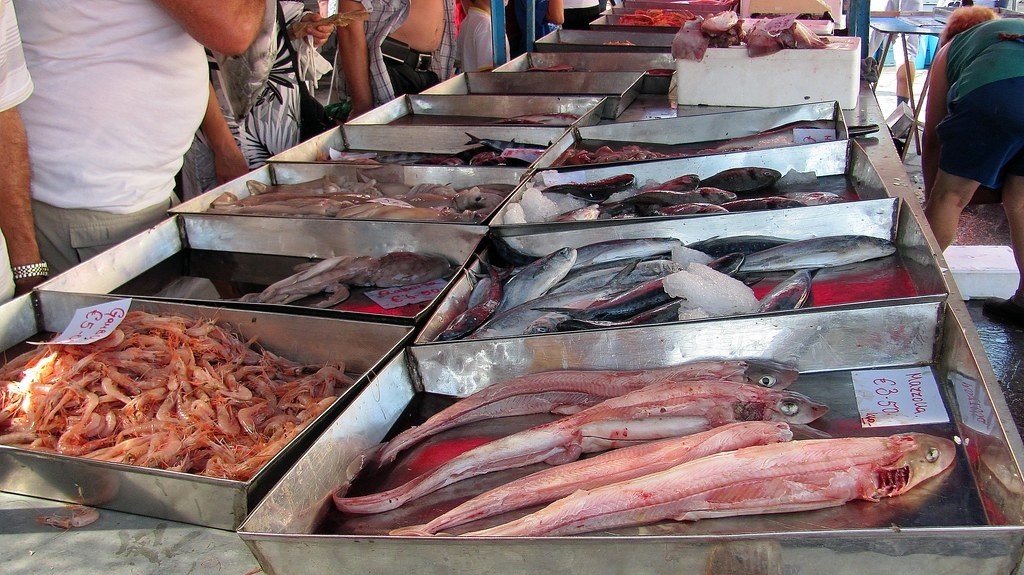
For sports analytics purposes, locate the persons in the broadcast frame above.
[0,0,607,306]
[870,0,923,107]
[921,5,1024,327]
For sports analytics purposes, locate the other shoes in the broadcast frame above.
[982,291,1024,330]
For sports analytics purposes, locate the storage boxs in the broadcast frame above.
[1,0,1024,575]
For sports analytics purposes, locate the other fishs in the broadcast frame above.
[478,111,580,125]
[431,165,900,342]
[326,360,956,538]
[464,129,554,155]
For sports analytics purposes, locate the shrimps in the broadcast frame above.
[1,309,355,478]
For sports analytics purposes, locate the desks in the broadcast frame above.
[869,17,1024,163]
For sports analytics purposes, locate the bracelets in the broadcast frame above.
[13,261,49,279]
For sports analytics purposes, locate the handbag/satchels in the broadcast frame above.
[298,80,332,143]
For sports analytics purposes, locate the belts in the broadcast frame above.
[380,39,434,73]
